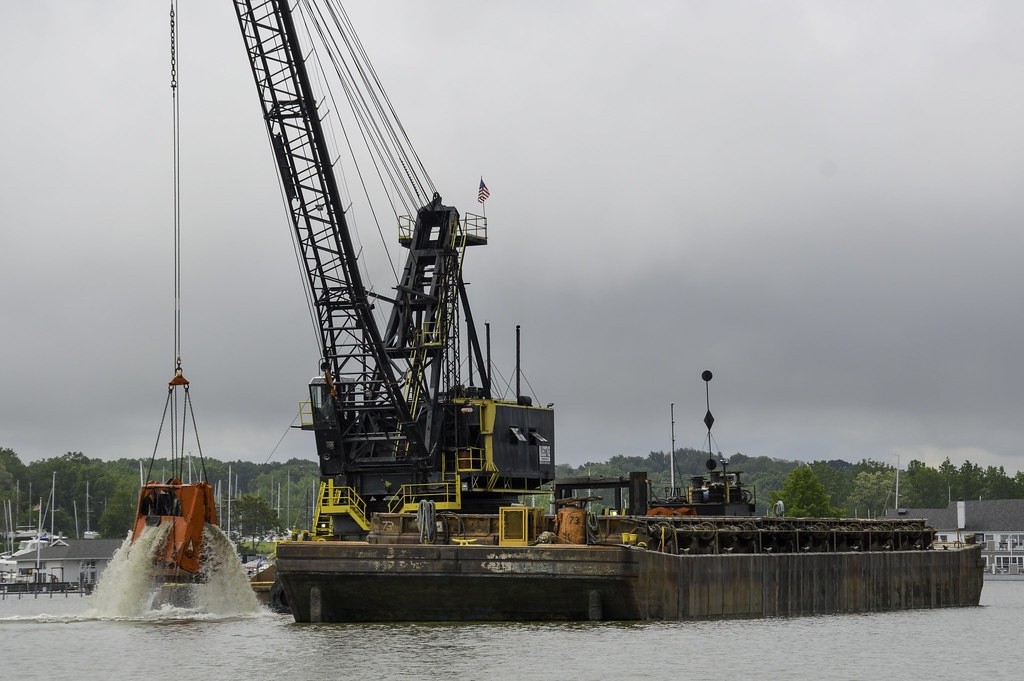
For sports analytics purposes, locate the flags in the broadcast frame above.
[478,181,490,203]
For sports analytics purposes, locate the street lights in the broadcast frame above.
[895,453,903,511]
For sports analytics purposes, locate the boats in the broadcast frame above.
[128,0,987,623]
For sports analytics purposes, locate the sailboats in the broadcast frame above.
[0,472,102,592]
